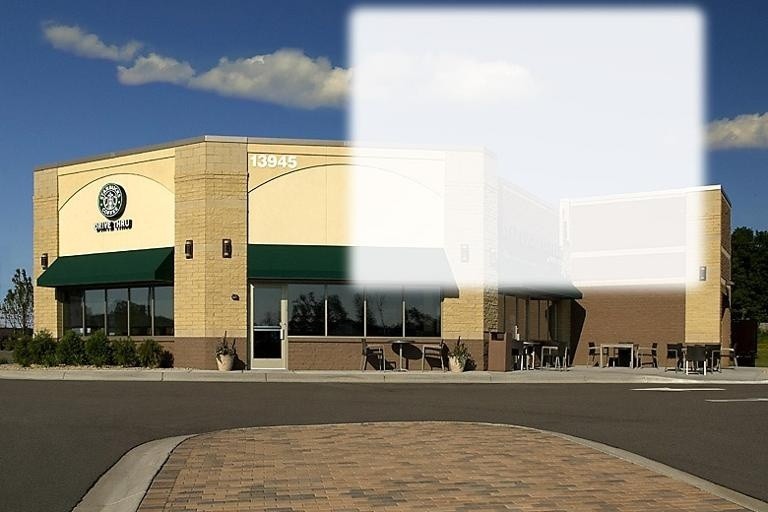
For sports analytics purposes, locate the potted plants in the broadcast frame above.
[214,331,237,371]
[447,336,471,372]
[109,335,165,368]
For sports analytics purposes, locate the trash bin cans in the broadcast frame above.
[488,330,513,372]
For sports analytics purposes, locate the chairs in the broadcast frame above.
[587,341,658,369]
[512,338,570,371]
[664,342,738,376]
[359,337,385,371]
[421,338,444,371]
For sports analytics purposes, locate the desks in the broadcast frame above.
[392,340,410,371]
